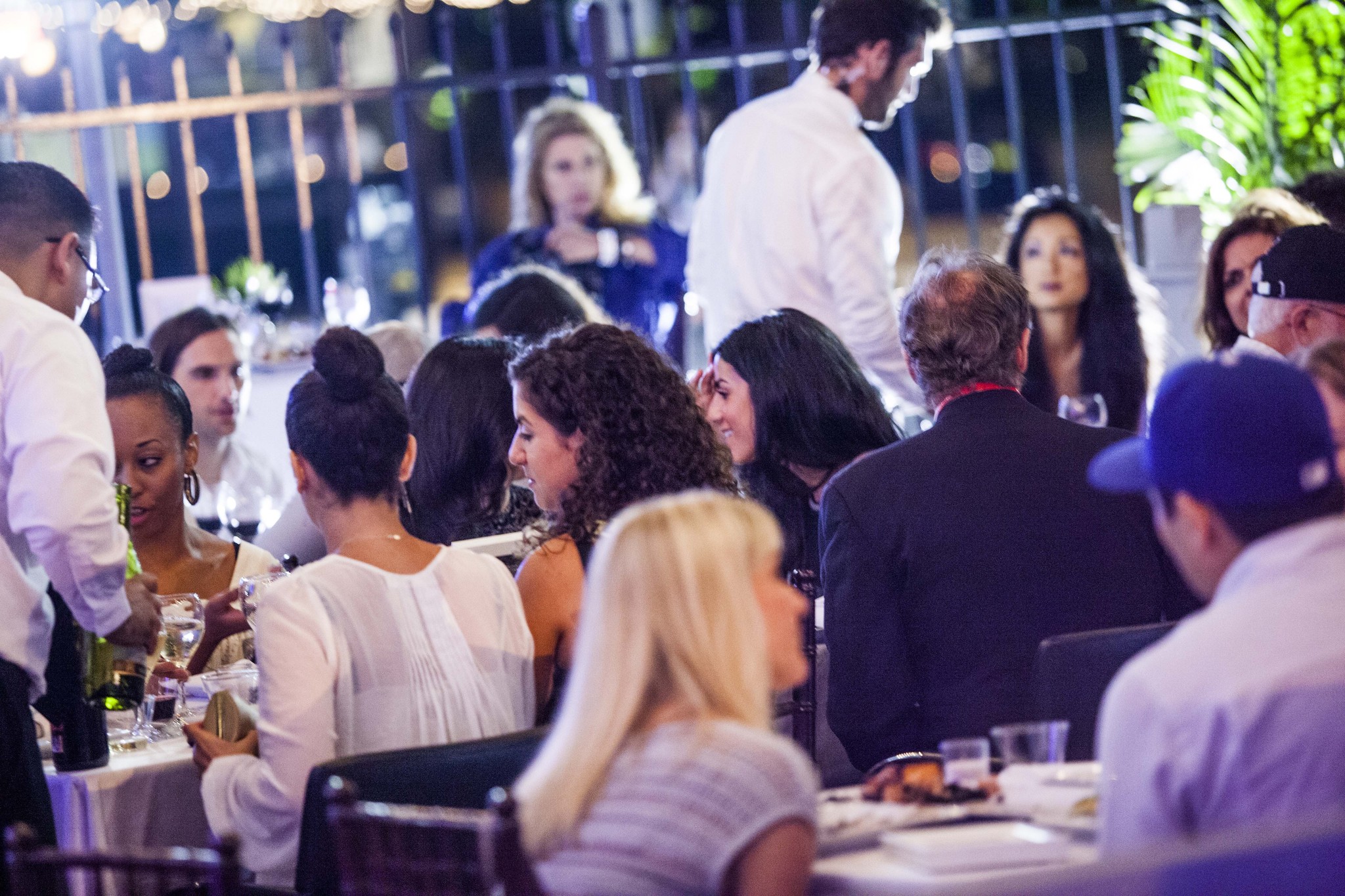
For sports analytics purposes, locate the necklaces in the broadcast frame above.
[365,535,401,540]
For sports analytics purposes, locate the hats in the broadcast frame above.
[1250,226,1345,322]
[1089,355,1337,503]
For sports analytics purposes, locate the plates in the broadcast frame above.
[186,671,207,696]
[998,760,1101,834]
[811,797,913,858]
[875,818,1064,873]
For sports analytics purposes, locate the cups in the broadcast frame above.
[240,570,289,633]
[993,722,1071,767]
[200,669,260,706]
[939,740,988,792]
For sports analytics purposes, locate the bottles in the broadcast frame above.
[83,482,146,710]
[44,581,110,772]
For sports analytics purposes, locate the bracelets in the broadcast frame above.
[596,228,619,268]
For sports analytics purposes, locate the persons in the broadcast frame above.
[689,307,901,596]
[398,336,539,546]
[686,0,950,422]
[1201,190,1330,364]
[145,306,284,531]
[182,326,536,886]
[1222,171,1345,482]
[470,96,694,372]
[514,485,820,896]
[815,250,1205,785]
[1003,189,1147,436]
[506,324,741,725]
[101,343,291,698]
[0,158,162,896]
[465,266,588,351]
[1089,358,1345,858]
[254,325,430,574]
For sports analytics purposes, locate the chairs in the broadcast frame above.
[0,522,1345,896]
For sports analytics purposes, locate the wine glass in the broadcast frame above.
[158,595,209,723]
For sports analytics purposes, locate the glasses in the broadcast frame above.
[46,236,109,304]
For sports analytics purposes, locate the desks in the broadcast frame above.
[26,692,211,896]
[234,351,315,531]
[807,759,1102,896]
